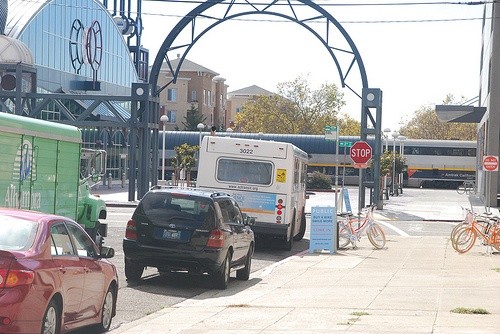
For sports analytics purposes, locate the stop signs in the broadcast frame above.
[351,142,372,166]
[482,155,499,172]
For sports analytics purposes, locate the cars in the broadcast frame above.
[0,207,119,334]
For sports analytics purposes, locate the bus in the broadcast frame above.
[198,136,308,251]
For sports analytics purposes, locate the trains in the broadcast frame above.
[80,128,477,188]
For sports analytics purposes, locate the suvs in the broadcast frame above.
[123,184,254,291]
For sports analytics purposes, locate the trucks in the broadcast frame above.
[0,112,108,240]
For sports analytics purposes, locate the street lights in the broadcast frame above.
[383,128,391,188]
[390,132,400,194]
[398,136,405,188]
[159,115,170,186]
[197,123,204,148]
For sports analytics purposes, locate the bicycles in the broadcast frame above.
[451,207,500,254]
[456,180,475,195]
[338,203,385,249]
[337,203,383,247]
